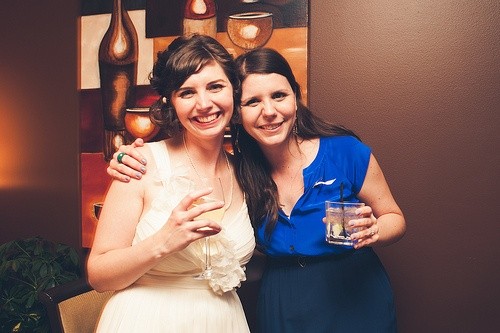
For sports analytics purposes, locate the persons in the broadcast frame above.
[106,47,405,333]
[87,32,256,333]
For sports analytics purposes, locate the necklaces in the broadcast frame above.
[181,130,234,212]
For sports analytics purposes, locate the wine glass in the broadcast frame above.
[188,178,227,280]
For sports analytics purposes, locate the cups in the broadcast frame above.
[325,200,365,247]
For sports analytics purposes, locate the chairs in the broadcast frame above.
[37,276,116,333]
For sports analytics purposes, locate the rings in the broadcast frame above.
[369,228,375,236]
[117,152,127,162]
